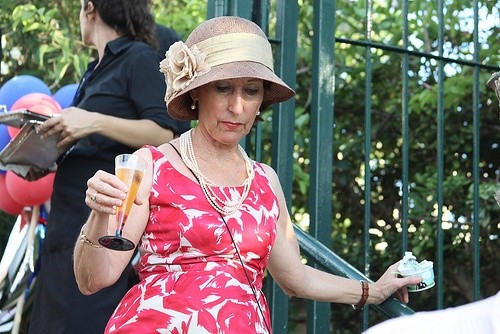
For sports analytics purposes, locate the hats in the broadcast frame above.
[158,15,295,120]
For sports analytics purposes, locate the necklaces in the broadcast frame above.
[180,127,255,215]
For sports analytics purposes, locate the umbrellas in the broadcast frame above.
[0,75,80,218]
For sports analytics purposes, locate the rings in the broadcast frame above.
[90,192,100,202]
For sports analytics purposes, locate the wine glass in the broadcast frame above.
[98,154,148,252]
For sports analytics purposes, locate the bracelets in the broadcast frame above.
[80,223,109,248]
[352,279,369,311]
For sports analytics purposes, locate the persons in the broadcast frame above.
[72,16,423,334]
[27,0,178,334]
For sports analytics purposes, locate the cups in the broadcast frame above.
[398,260,435,292]
